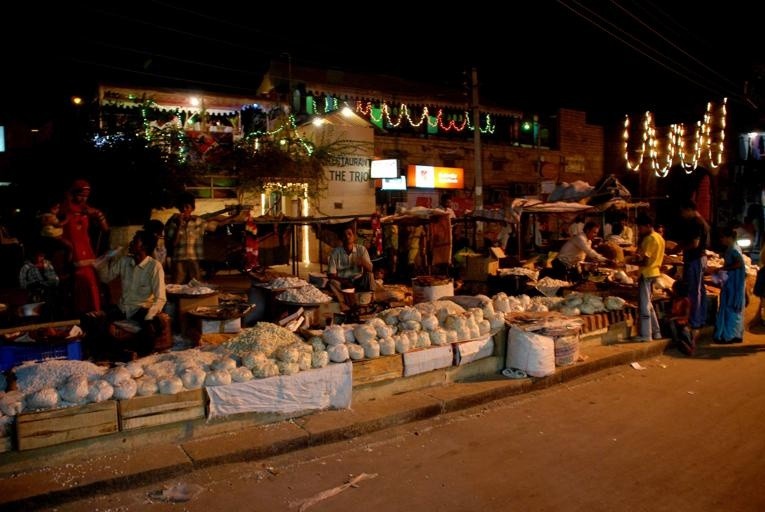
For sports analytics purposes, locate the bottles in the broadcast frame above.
[92,245,126,272]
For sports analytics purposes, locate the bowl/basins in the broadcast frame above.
[340,287,377,307]
[579,261,601,273]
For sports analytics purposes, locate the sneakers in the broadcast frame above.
[654,332,662,338]
[633,335,652,342]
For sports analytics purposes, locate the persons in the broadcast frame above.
[327,226,377,310]
[79,229,167,359]
[18,246,61,290]
[59,178,110,311]
[164,192,242,286]
[550,197,765,344]
[143,219,166,267]
[42,198,64,244]
